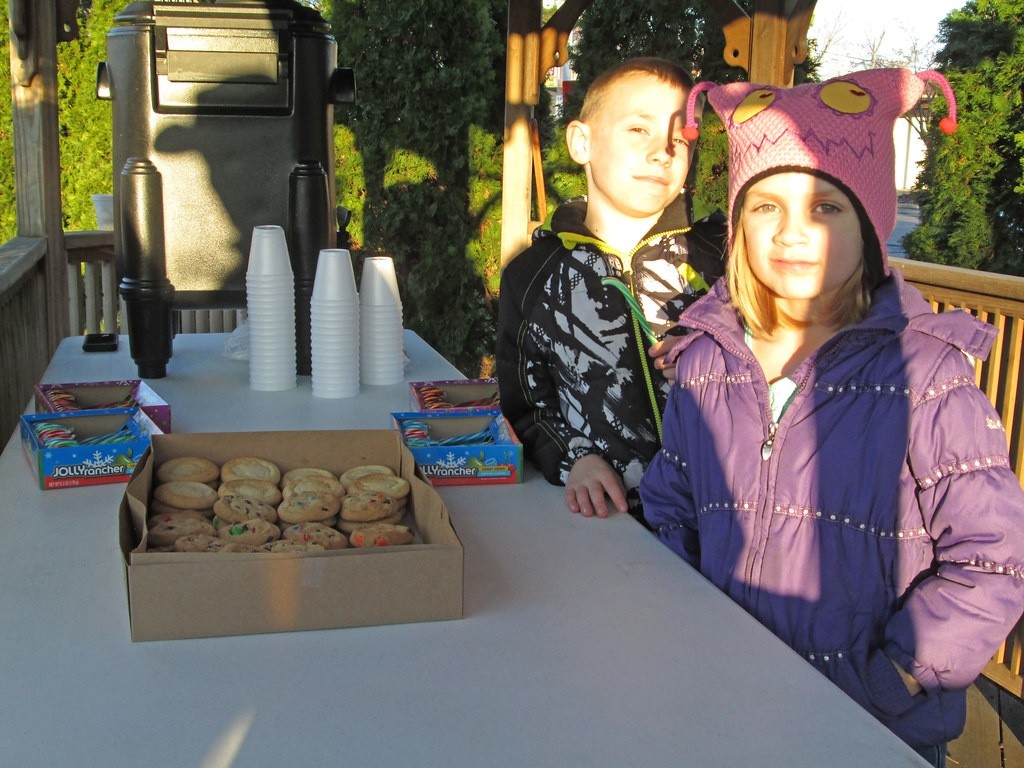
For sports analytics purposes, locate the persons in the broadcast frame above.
[637,68,1024,767]
[495,55,725,521]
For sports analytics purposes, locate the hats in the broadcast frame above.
[682,70,956,279]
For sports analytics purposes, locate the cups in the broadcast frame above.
[91,194,114,231]
[310,249,403,398]
[246,225,297,391]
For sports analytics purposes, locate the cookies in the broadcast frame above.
[143,456,415,552]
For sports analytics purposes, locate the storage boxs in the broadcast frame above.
[409,379,501,413]
[117,429,465,640]
[17,407,168,490]
[390,412,525,486]
[33,379,171,435]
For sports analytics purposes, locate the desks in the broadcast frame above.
[0,292,934,768]
